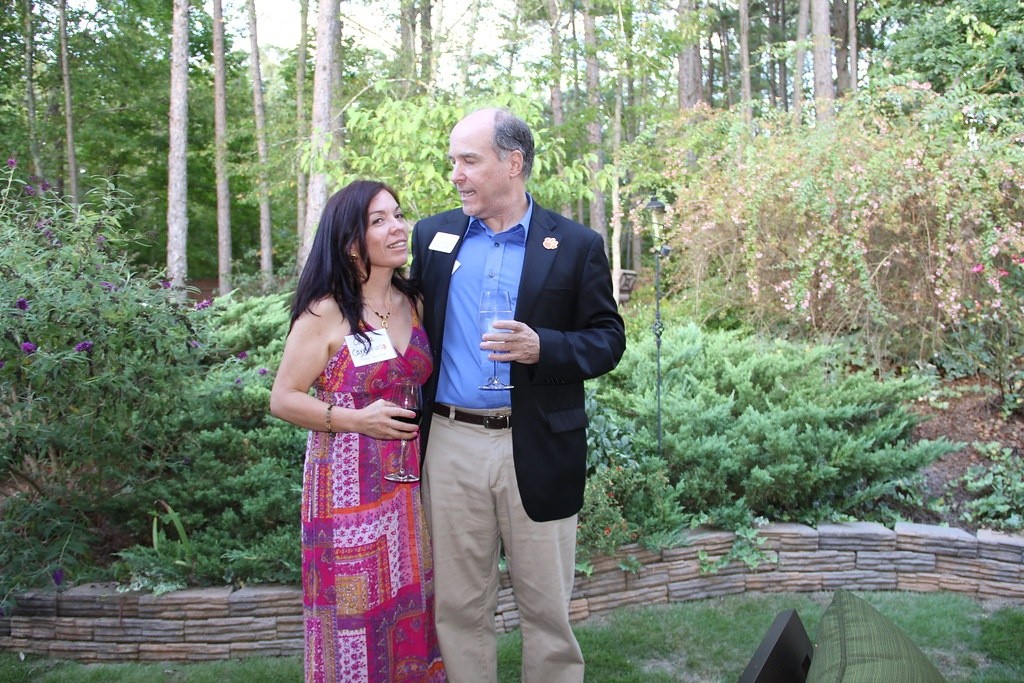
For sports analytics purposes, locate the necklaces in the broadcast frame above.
[359,289,393,329]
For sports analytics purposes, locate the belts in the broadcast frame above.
[433,402,512,429]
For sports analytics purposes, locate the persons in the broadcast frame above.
[268,180,451,683]
[407,107,628,683]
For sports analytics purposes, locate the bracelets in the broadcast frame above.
[326,403,338,438]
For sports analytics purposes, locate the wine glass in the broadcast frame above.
[479,290,515,391]
[383,381,422,483]
